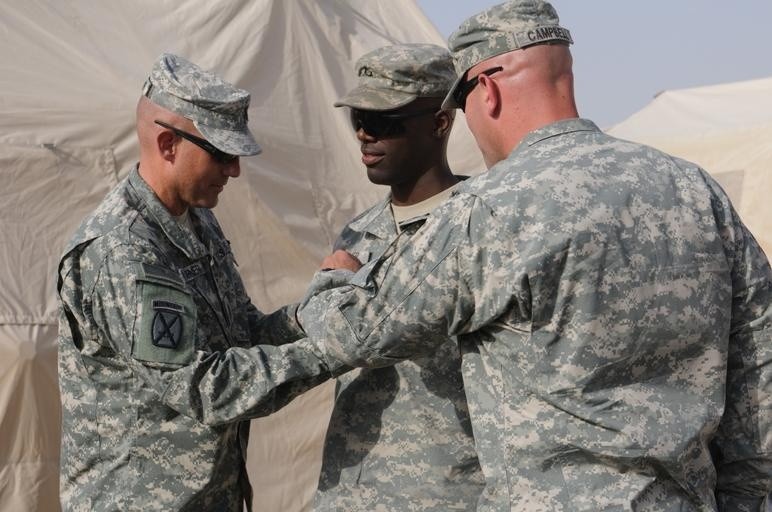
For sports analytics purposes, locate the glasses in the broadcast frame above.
[155,119,240,162]
[350,105,440,137]
[453,65,503,109]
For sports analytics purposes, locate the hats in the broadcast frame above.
[436,0,562,111]
[334,42,456,113]
[141,51,263,156]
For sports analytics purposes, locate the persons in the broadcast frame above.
[297,0,772,512]
[54,54,333,512]
[300,43,486,512]
